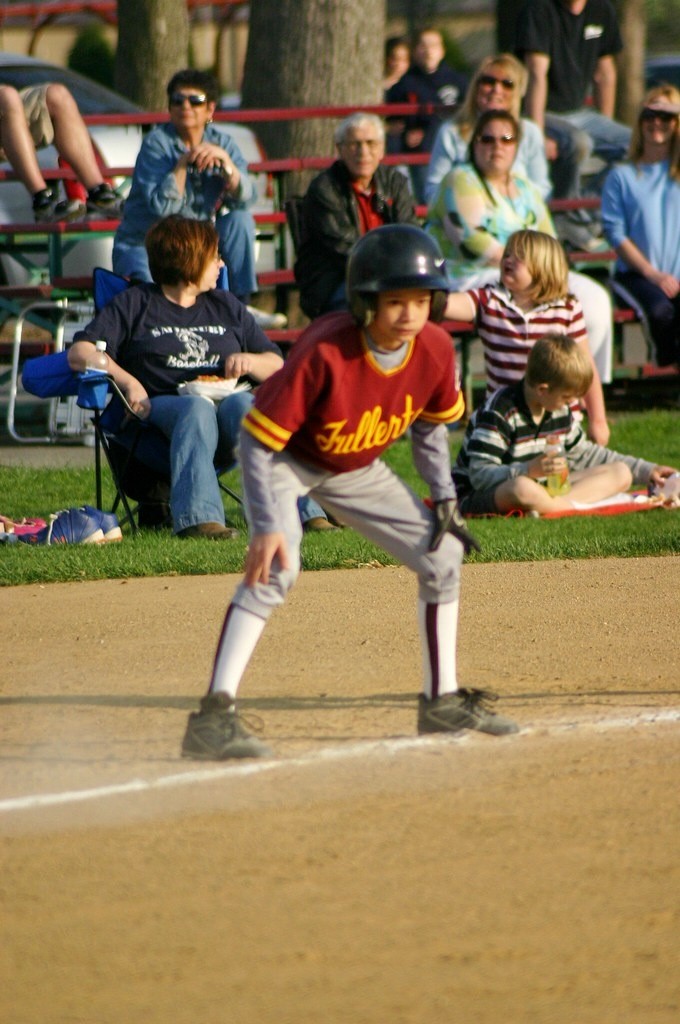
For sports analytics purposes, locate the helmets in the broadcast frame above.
[345,223,450,329]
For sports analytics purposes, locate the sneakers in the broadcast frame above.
[302,518,341,531]
[1,515,46,543]
[416,688,519,737]
[245,305,288,330]
[31,184,85,223]
[85,181,125,221]
[180,691,281,760]
[19,504,123,546]
[182,522,235,539]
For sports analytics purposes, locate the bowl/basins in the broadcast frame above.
[177,379,237,399]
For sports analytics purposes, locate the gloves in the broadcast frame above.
[428,496,482,558]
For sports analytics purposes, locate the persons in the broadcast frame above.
[447,228,611,449]
[519,0,624,223]
[67,216,344,540]
[450,335,678,514]
[381,29,467,156]
[111,70,288,328]
[425,53,558,289]
[180,225,518,760]
[296,113,448,320]
[599,85,680,369]
[0,82,121,209]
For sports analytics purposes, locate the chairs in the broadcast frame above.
[22,267,246,533]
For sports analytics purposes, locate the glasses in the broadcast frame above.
[169,93,207,107]
[474,134,517,145]
[478,74,515,88]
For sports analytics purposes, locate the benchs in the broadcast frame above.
[0,103,637,425]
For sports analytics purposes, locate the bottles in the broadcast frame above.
[653,472,680,499]
[545,435,571,498]
[85,341,109,376]
